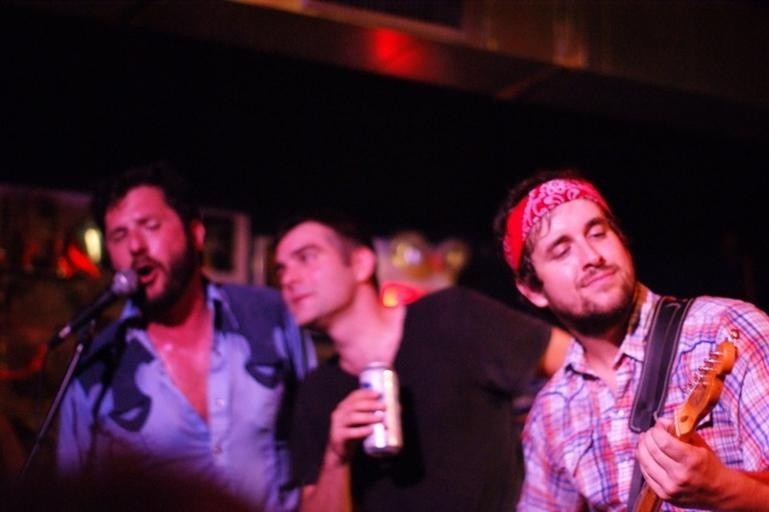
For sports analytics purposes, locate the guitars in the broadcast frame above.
[633,342,735,510]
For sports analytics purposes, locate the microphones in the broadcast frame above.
[44,266,141,348]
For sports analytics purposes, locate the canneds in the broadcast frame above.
[358,361,405,459]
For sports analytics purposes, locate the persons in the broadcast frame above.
[490,167,768,512]
[46,165,321,512]
[265,215,571,512]
